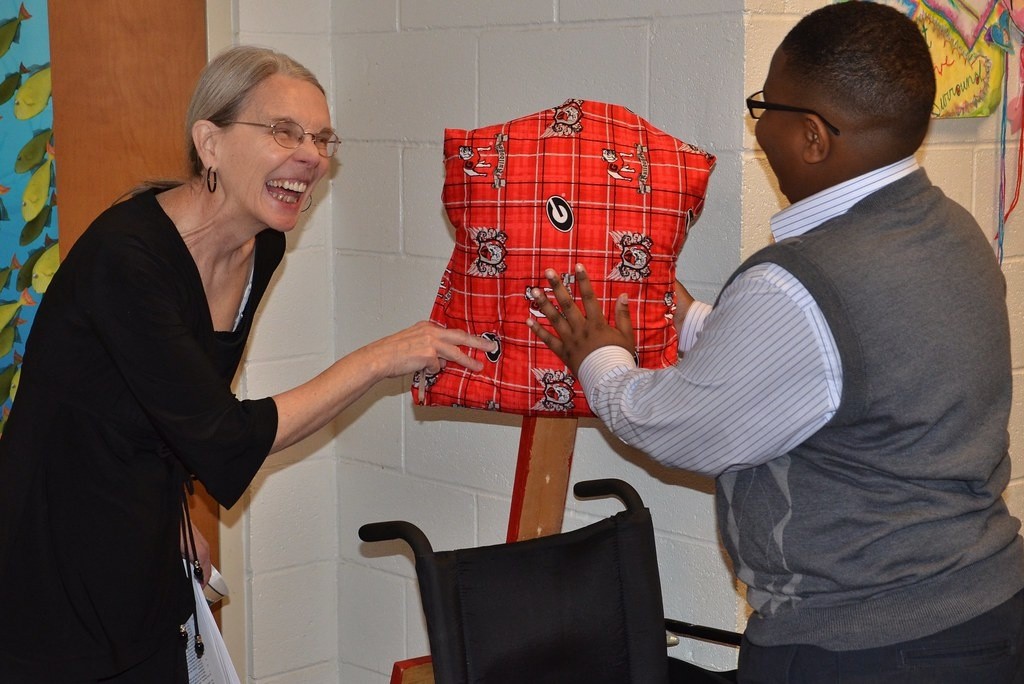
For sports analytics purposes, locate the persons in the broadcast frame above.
[529,1,1024,684]
[0,46,496,683]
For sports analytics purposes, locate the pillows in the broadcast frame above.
[411,99,717,417]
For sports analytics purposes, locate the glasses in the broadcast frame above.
[746,90,841,135]
[219,119,342,159]
